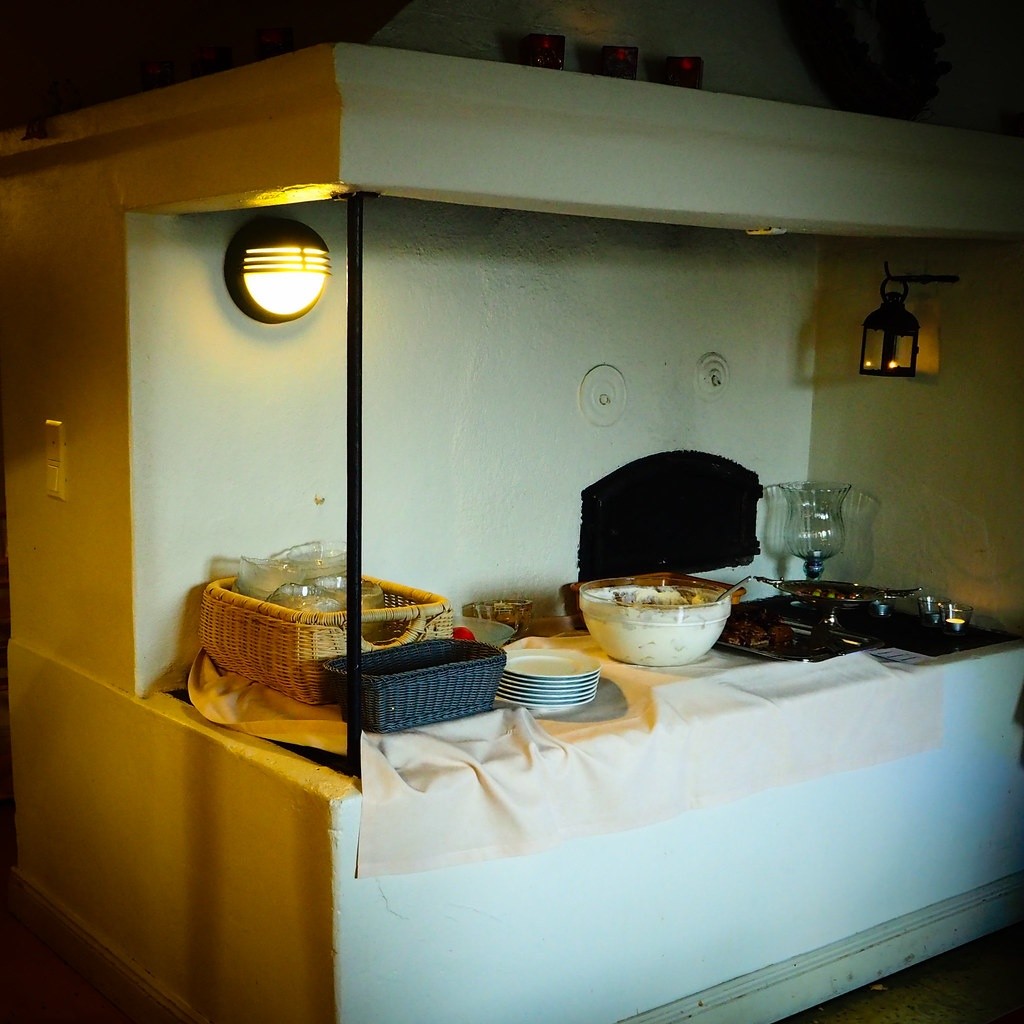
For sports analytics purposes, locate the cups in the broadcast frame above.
[939,602,972,636]
[869,588,896,618]
[918,596,950,627]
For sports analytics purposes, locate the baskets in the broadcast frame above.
[321,639,506,734]
[198,575,453,706]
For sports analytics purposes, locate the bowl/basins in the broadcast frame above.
[580,577,731,666]
[453,616,514,647]
[473,600,533,639]
[232,542,384,641]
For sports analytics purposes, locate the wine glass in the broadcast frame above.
[780,482,851,609]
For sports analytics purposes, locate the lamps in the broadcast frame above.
[858,261,960,379]
[221,216,333,325]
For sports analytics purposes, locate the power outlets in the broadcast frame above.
[43,420,65,462]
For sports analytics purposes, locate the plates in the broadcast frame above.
[497,649,601,708]
[718,618,884,664]
[754,576,921,610]
